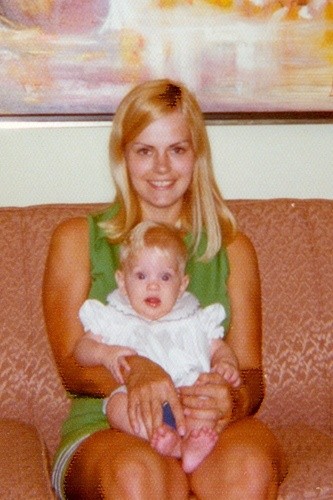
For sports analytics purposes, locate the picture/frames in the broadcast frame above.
[0,0,333,120]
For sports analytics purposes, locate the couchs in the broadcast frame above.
[0,198,333,500]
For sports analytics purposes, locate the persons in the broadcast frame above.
[42,78,281,500]
[72,225,241,473]
